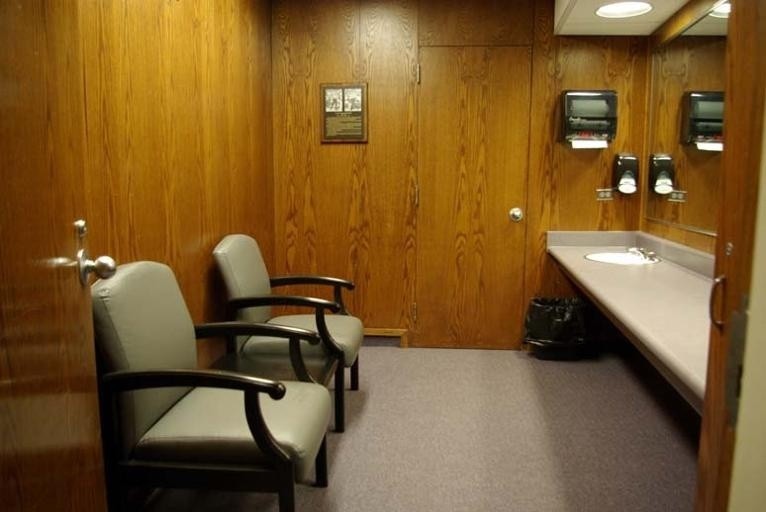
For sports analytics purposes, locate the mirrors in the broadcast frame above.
[643,1,732,256]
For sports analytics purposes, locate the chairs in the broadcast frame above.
[209,233,363,432]
[91,258,337,507]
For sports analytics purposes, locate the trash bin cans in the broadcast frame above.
[529,297,593,361]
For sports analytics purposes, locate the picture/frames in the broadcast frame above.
[319,79,368,144]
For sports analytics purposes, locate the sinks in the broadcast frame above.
[585,251,649,266]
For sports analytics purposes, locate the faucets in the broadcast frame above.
[638,247,657,262]
